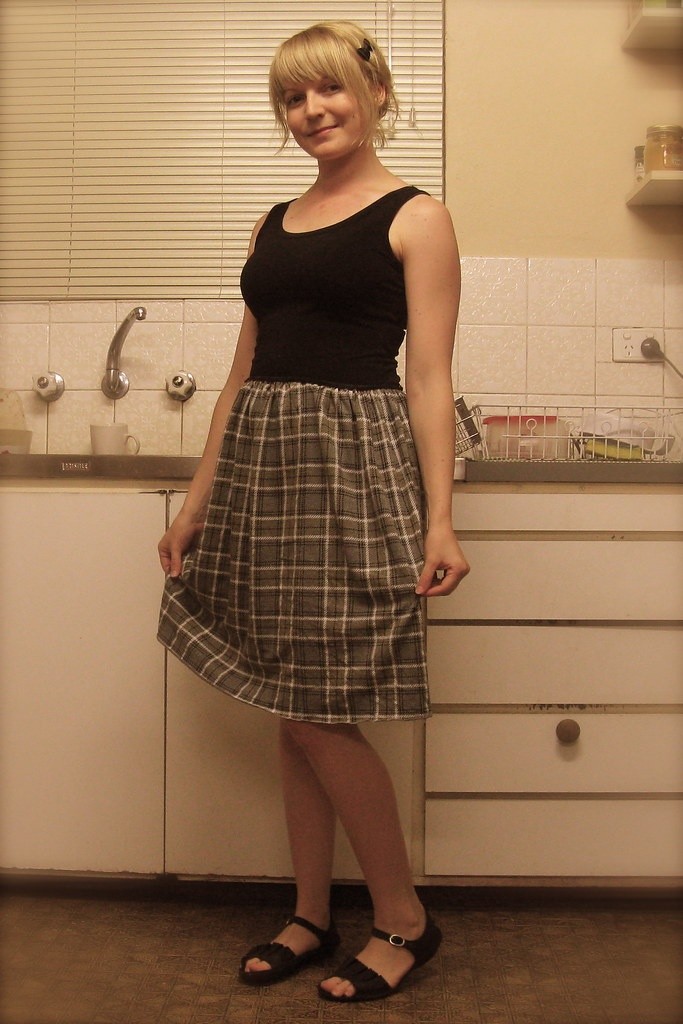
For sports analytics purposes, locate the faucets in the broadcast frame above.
[104,305,148,392]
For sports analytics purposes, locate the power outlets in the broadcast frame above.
[612,328,665,364]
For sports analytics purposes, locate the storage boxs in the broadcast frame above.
[481,415,556,460]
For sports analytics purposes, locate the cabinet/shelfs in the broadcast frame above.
[418,483,683,888]
[619,8,682,206]
[0,479,416,885]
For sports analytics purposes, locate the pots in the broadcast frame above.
[578,412,676,455]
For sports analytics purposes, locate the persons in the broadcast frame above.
[155,21,460,1005]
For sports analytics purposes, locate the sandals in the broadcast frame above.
[239,914,341,987]
[317,904,445,1000]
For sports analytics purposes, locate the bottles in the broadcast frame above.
[633,123,683,184]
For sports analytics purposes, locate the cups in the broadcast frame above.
[90,423,141,454]
[0,429,33,454]
